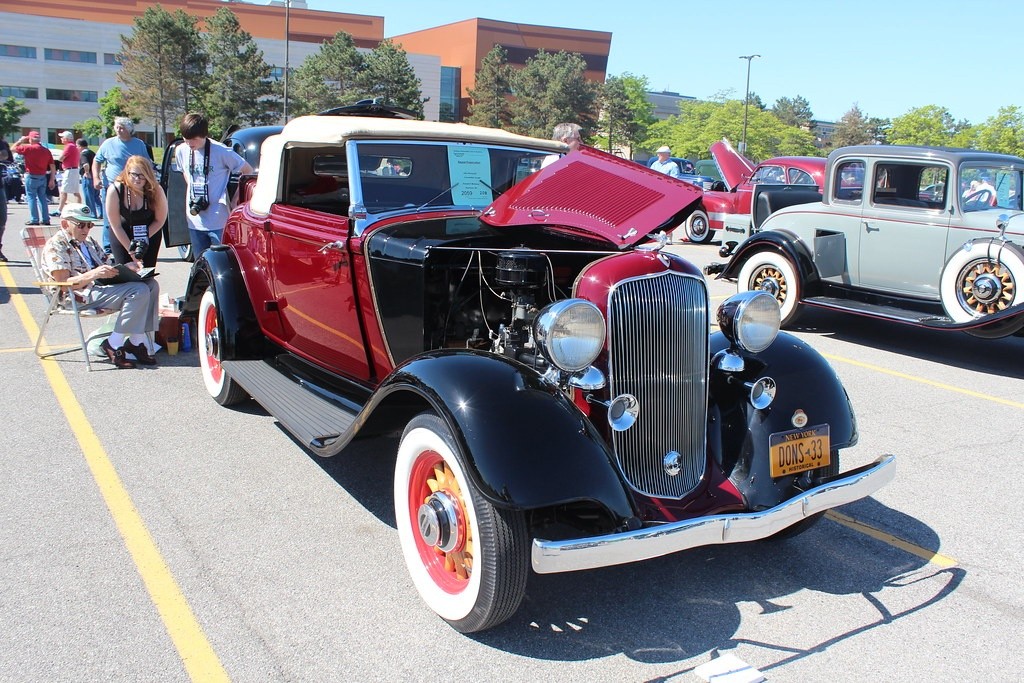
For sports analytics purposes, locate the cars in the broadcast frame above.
[703,145,1024,339]
[924,183,945,203]
[635,136,931,244]
[160,94,426,263]
[179,115,896,633]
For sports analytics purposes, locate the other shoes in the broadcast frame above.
[108,252,115,260]
[0,252,8,262]
[39,221,50,225]
[48,210,61,216]
[666,239,672,245]
[25,220,39,225]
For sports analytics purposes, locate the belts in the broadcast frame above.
[67,167,78,169]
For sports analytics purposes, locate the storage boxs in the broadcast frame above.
[156,309,183,347]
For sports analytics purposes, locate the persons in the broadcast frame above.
[92,115,151,258]
[10,130,55,226]
[0,132,14,261]
[75,138,103,219]
[962,171,996,207]
[539,123,584,170]
[650,145,680,244]
[381,158,406,176]
[41,202,160,368]
[48,130,83,217]
[106,154,169,279]
[175,113,252,262]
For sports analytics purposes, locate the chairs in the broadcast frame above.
[20,225,155,371]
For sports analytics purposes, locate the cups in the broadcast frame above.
[166,338,179,356]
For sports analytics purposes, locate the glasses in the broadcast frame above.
[69,221,94,229]
[128,172,146,180]
[570,137,582,141]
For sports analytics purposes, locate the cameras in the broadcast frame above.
[189,197,208,216]
[129,240,148,259]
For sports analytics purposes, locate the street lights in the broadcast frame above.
[739,54,761,156]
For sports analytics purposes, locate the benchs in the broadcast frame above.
[293,181,447,215]
[876,196,930,208]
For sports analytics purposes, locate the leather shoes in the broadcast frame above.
[124,338,157,364]
[100,339,137,369]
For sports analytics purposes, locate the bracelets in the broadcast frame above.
[128,252,135,255]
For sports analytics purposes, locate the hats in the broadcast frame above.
[29,131,41,139]
[655,146,671,153]
[116,117,135,135]
[61,203,99,221]
[58,131,74,139]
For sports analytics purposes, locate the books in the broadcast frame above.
[96,262,160,286]
